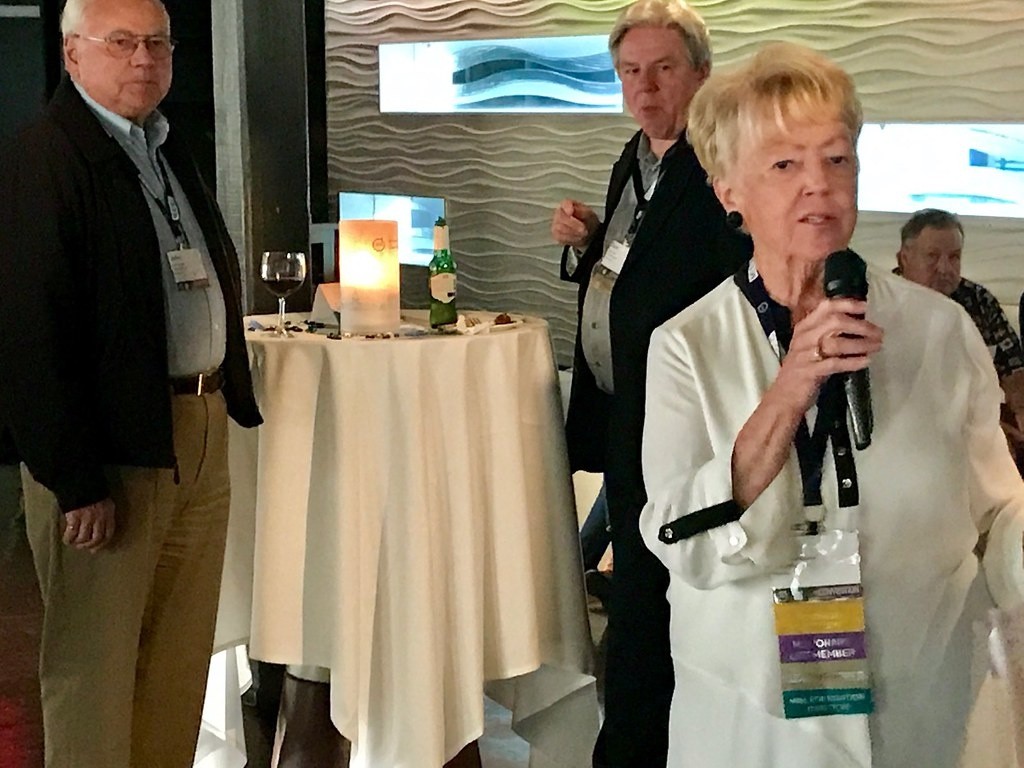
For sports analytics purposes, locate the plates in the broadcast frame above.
[468,319,523,332]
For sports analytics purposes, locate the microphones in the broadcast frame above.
[825,249,875,450]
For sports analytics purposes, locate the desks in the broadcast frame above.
[193,309,597,768]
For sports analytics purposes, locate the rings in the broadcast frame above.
[66,524,79,530]
[813,337,825,360]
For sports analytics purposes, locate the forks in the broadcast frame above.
[462,313,481,327]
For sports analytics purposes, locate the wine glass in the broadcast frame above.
[260,251,306,338]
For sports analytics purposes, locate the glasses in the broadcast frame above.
[71,29,175,59]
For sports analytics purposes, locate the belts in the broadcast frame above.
[169,369,226,397]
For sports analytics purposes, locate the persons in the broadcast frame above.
[886,208,1023,471]
[554,0,755,768]
[638,39,1024,768]
[0,0,265,766]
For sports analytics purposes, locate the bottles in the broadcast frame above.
[428,216,458,328]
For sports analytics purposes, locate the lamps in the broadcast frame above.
[338,219,401,337]
